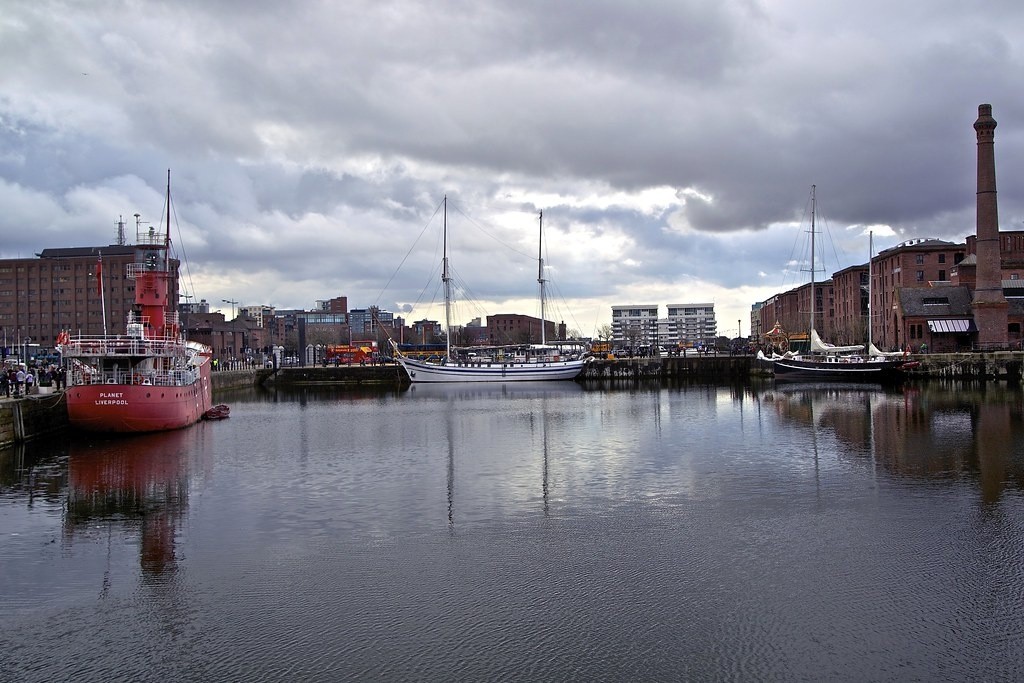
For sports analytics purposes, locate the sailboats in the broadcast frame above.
[369,193,593,382]
[753,185,921,378]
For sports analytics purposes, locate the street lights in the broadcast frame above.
[179,294,193,341]
[223,300,239,337]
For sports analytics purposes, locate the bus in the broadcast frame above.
[327,340,380,364]
[393,343,448,362]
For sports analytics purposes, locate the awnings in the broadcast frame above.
[927,319,979,332]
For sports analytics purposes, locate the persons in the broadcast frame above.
[250,356,255,369]
[210,359,230,373]
[731,344,788,356]
[0,360,66,398]
[360,355,384,366]
[628,345,681,357]
[336,354,341,367]
[698,344,720,356]
[322,356,327,367]
[920,342,927,354]
[266,355,273,368]
[899,342,911,352]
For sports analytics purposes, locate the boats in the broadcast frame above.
[206,403,230,421]
[60,167,212,434]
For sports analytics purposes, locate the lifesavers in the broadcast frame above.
[132,372,144,385]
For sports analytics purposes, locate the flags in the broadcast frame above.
[96,258,101,296]
